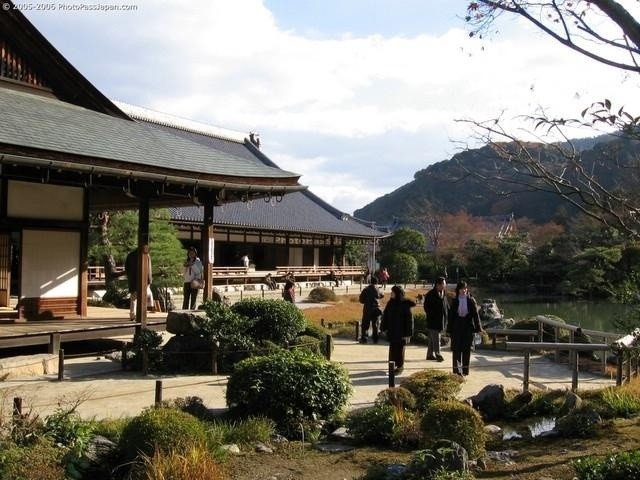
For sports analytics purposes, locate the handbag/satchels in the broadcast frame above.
[191,279,205,289]
[147,284,154,307]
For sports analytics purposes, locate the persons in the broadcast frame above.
[424,276,450,362]
[448,280,485,378]
[264,273,276,291]
[182,247,202,312]
[359,276,385,345]
[125,251,154,321]
[283,272,297,303]
[378,267,390,290]
[330,270,339,287]
[380,285,416,376]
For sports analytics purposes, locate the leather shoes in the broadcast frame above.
[427,354,444,362]
[453,371,468,377]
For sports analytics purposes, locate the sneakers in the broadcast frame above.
[394,367,404,376]
